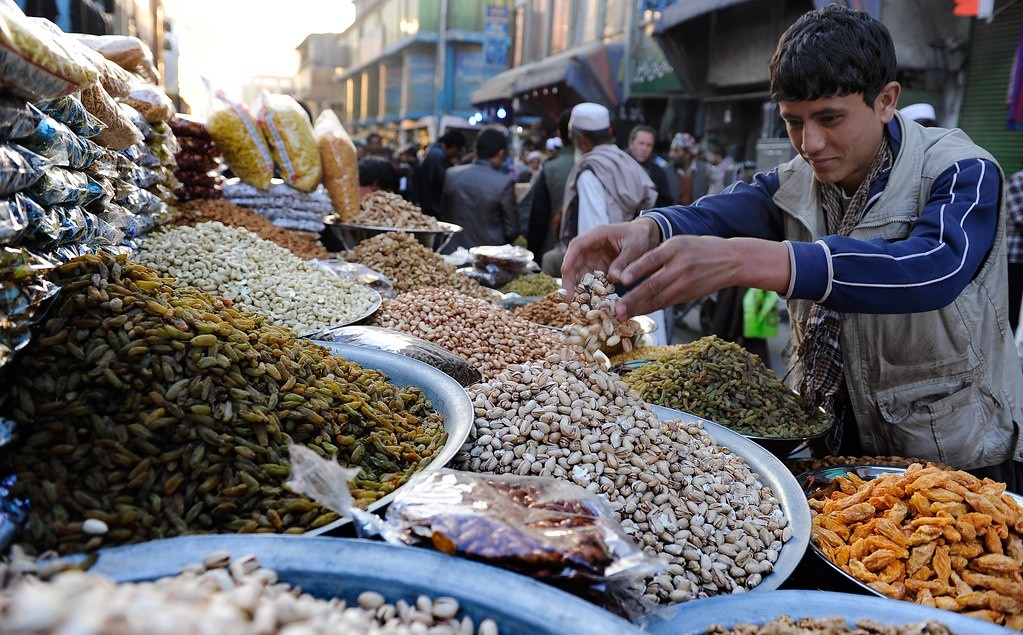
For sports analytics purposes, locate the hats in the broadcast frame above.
[671,133,696,150]
[546,137,563,151]
[899,103,936,123]
[528,151,542,161]
[569,102,610,131]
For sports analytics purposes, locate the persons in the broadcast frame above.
[1003,171,1023,341]
[560,0,1023,488]
[899,103,938,128]
[556,102,668,347]
[355,110,771,371]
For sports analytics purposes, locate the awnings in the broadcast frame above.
[470,43,626,110]
[652,0,881,93]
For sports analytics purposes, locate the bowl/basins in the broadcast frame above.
[37,285,1023,635]
[325,214,462,254]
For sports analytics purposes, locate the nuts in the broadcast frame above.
[0,195,794,634]
